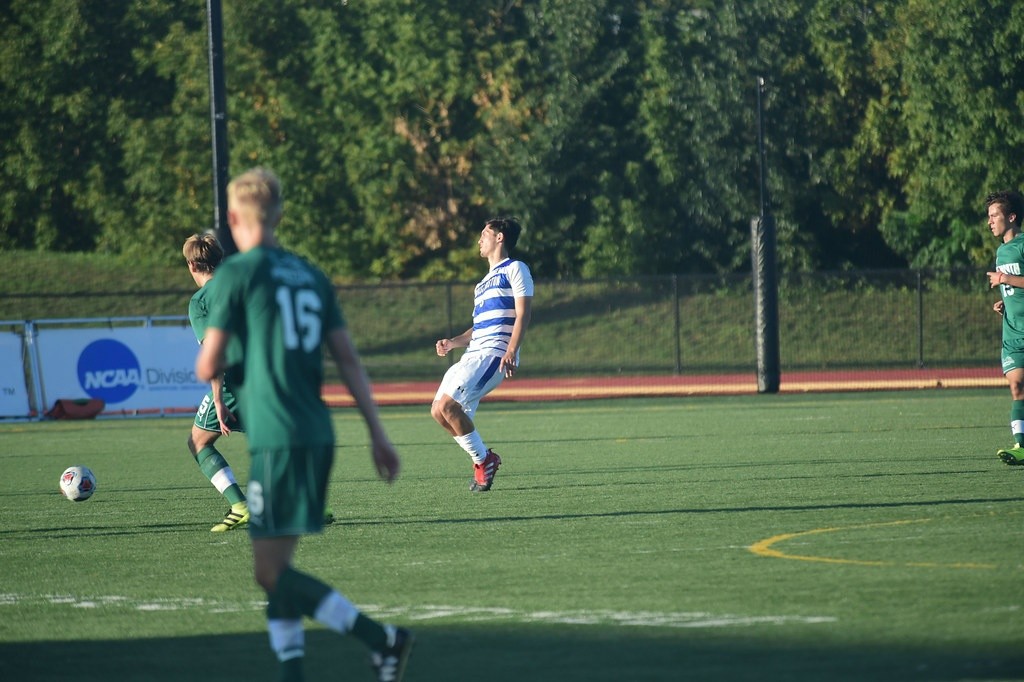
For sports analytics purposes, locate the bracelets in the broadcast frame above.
[999,274,1004,285]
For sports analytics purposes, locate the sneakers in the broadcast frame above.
[368,622,415,682]
[470,447,502,492]
[323,508,333,520]
[211,506,250,533]
[996,442,1024,465]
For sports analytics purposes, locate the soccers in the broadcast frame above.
[60,466,96,502]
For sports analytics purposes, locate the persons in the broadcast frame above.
[982,191,1024,466]
[431,216,534,491]
[182,168,416,682]
